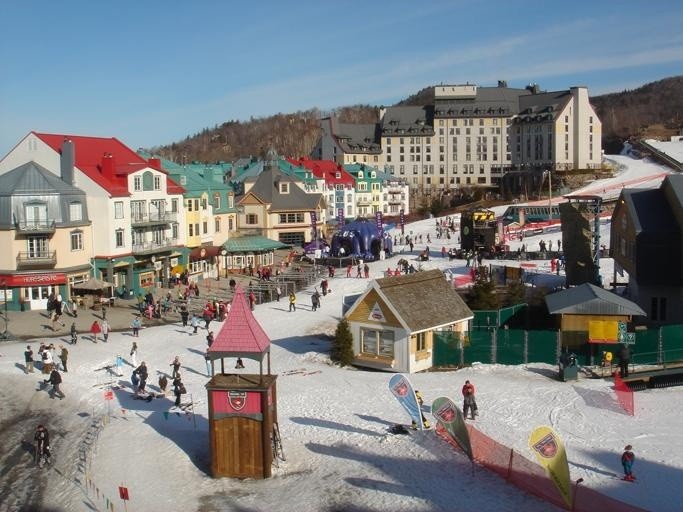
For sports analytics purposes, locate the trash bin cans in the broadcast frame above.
[168,280,175,289]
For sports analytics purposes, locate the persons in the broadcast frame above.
[412,391,431,431]
[621,445,635,482]
[463,387,475,421]
[615,345,629,379]
[462,380,479,417]
[33,425,51,469]
[386,216,562,282]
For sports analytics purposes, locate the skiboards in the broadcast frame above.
[608,473,640,483]
[37,432,45,469]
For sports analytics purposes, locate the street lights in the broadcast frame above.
[543,169,551,225]
[2,280,10,337]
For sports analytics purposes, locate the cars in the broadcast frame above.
[459,249,473,259]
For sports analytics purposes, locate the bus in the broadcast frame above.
[502,205,559,222]
